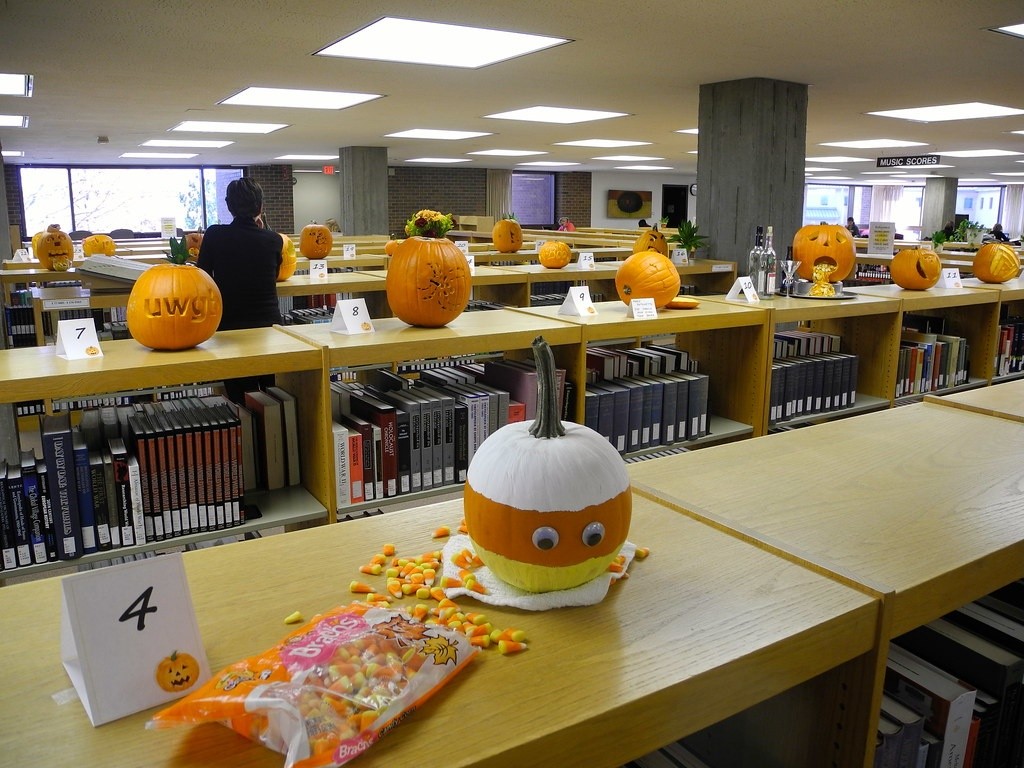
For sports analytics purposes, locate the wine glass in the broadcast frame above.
[779,260,803,300]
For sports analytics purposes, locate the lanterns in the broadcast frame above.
[125,264,223,351]
[465,418,632,594]
[387,235,473,326]
[890,243,1020,289]
[30,219,857,310]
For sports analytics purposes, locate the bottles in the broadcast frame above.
[780,246,794,294]
[747,225,766,300]
[759,225,776,300]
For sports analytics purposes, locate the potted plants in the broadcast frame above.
[932,228,947,253]
[949,219,990,249]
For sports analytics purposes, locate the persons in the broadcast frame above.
[557,217,576,232]
[638,219,651,227]
[847,217,1009,241]
[196,177,284,394]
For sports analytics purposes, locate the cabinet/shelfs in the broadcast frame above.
[0,216,1024,768]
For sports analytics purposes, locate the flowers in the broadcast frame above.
[405,209,454,238]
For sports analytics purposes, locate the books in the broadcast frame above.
[895,317,1024,398]
[3,281,127,348]
[0,384,301,571]
[332,347,709,524]
[278,260,604,322]
[768,330,859,434]
[618,577,1024,768]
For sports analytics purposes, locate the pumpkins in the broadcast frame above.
[385,234,401,255]
[492,215,523,252]
[616,251,680,308]
[465,335,631,594]
[539,240,572,268]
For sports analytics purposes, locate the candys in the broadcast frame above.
[283,514,648,757]
